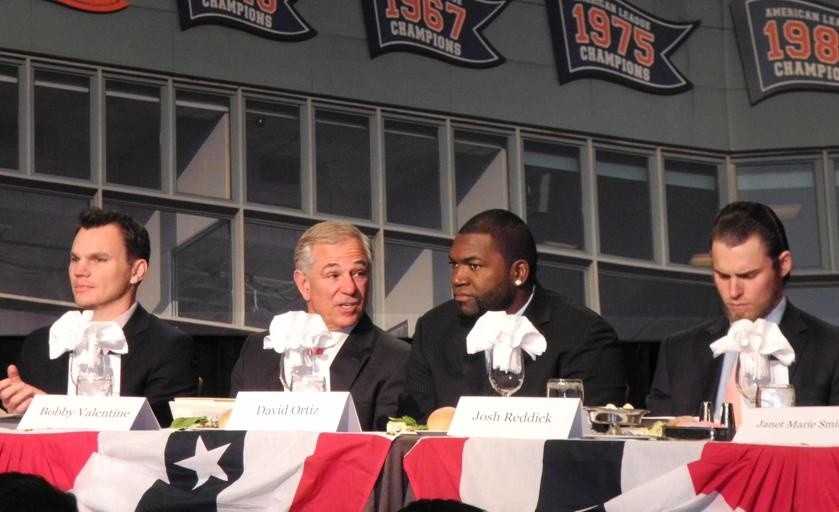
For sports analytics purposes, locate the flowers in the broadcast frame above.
[387,414,427,434]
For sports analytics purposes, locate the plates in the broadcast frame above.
[415,428,451,436]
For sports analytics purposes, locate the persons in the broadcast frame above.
[229,218,410,431]
[643,201,839,424]
[397,207,625,433]
[0,206,195,429]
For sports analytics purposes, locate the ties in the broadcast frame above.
[725,361,741,434]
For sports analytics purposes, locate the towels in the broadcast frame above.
[466,311,547,374]
[48,311,129,368]
[262,309,338,369]
[710,318,796,379]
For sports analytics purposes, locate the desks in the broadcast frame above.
[0,430,839,512]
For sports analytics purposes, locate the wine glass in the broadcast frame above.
[484,340,526,396]
[279,343,315,392]
[70,342,106,388]
[735,350,772,406]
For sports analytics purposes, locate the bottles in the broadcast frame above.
[718,401,737,439]
[698,400,715,423]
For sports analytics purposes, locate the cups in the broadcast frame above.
[545,377,584,402]
[288,375,326,391]
[75,374,113,395]
[755,383,796,408]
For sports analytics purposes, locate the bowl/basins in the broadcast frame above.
[169,396,234,421]
